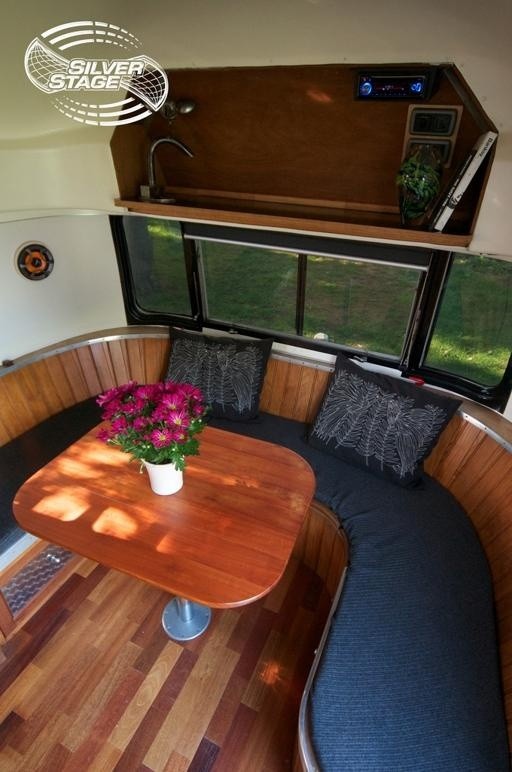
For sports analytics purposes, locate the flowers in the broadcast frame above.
[96,380,212,471]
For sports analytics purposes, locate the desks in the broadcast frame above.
[11,420,316,642]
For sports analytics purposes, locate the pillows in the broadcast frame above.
[302,351,463,491]
[164,328,273,426]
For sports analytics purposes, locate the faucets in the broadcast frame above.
[138,137,196,197]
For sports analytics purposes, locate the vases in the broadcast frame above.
[395,145,444,229]
[140,456,183,495]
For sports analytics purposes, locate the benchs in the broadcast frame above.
[0,327,512,772]
